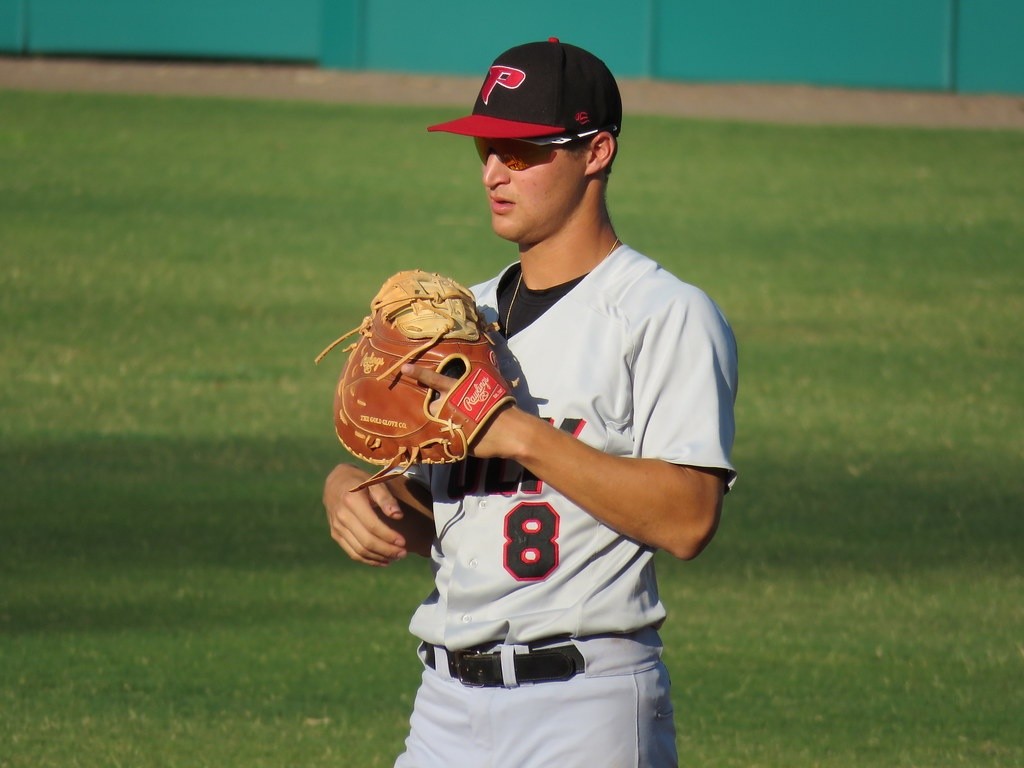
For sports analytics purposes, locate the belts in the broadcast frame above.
[423,643,584,688]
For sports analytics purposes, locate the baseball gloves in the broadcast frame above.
[331,269,519,469]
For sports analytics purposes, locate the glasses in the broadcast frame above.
[473,130,619,171]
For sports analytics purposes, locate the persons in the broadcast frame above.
[322,37,739,768]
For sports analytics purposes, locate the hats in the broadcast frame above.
[425,36,623,149]
